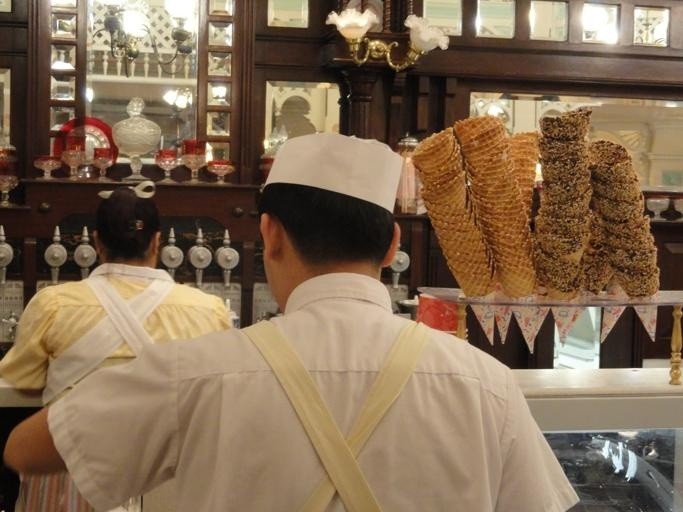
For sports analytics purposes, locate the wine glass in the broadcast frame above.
[672,196,683,222]
[0,172,19,206]
[62,136,85,180]
[155,150,178,184]
[646,195,670,221]
[181,141,207,183]
[207,161,235,185]
[34,156,63,180]
[93,148,114,181]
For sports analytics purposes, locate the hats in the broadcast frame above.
[261,132,405,215]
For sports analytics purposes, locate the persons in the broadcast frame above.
[0,188,236,509]
[4,132,583,511]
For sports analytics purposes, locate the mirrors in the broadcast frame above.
[86,0,199,164]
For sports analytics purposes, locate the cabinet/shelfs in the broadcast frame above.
[511,368,683,512]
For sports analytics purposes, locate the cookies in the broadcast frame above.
[413,108,660,354]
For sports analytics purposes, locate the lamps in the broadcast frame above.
[325,8,449,72]
[93,1,198,77]
[162,87,193,123]
